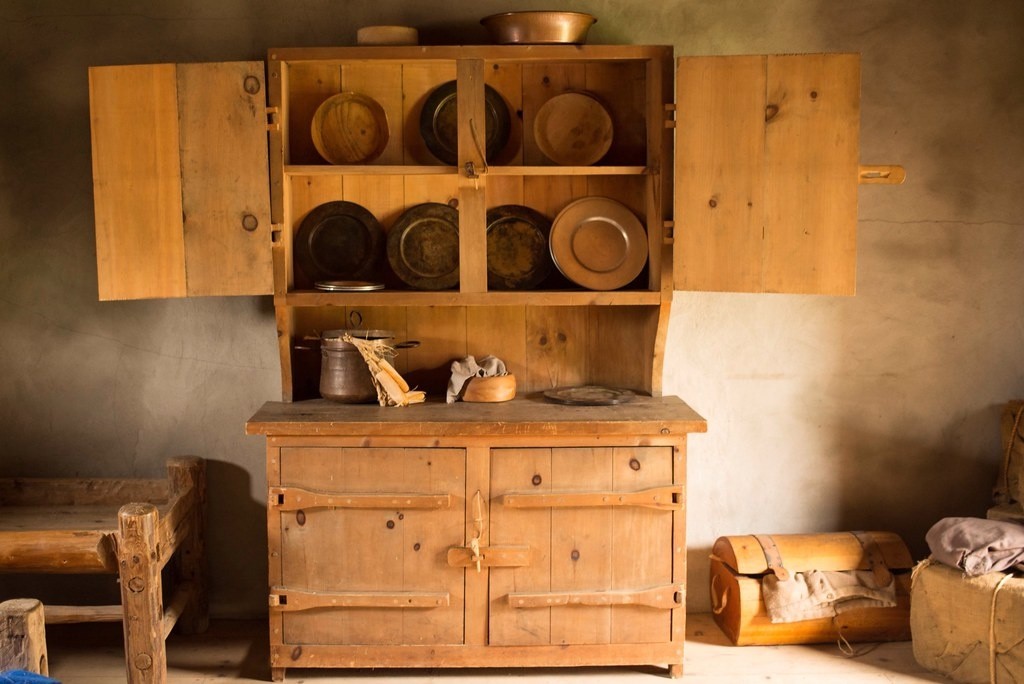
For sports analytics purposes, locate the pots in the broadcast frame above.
[294,311,421,404]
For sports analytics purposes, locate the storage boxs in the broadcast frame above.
[711,531,917,646]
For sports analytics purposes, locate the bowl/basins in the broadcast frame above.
[461,370,516,403]
[481,11,599,44]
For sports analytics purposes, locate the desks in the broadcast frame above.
[0,455,210,684]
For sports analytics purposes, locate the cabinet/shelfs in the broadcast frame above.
[88,44,864,679]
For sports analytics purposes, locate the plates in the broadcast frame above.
[311,91,389,166]
[419,80,510,165]
[387,202,459,293]
[544,386,634,405]
[487,205,550,290]
[315,280,385,292]
[297,200,383,286]
[549,196,649,289]
[534,92,614,166]
